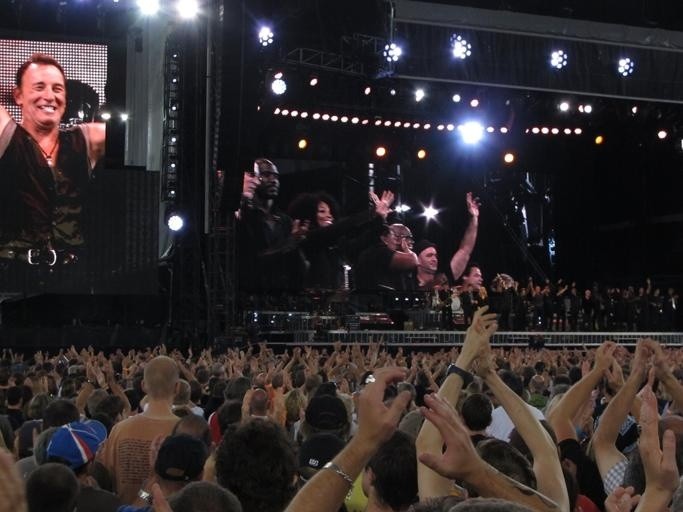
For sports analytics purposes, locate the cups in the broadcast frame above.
[397,232,416,240]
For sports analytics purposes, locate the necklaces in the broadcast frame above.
[37,134,58,166]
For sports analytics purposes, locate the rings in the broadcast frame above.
[364,375,376,383]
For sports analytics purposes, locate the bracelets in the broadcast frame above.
[138,489,153,504]
[87,380,95,385]
[323,462,355,490]
[480,368,495,377]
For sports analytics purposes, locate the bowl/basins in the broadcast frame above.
[37,134,58,166]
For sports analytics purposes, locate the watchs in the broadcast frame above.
[445,364,474,390]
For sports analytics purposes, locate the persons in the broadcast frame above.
[376,190,483,286]
[233,156,307,292]
[0,53,106,291]
[0,306,683,512]
[423,276,682,332]
[292,189,394,287]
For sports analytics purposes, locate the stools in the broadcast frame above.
[4,246,71,267]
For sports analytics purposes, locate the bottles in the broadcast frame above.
[417,239,436,254]
[299,430,346,474]
[304,393,346,431]
[154,416,214,484]
[44,416,108,467]
